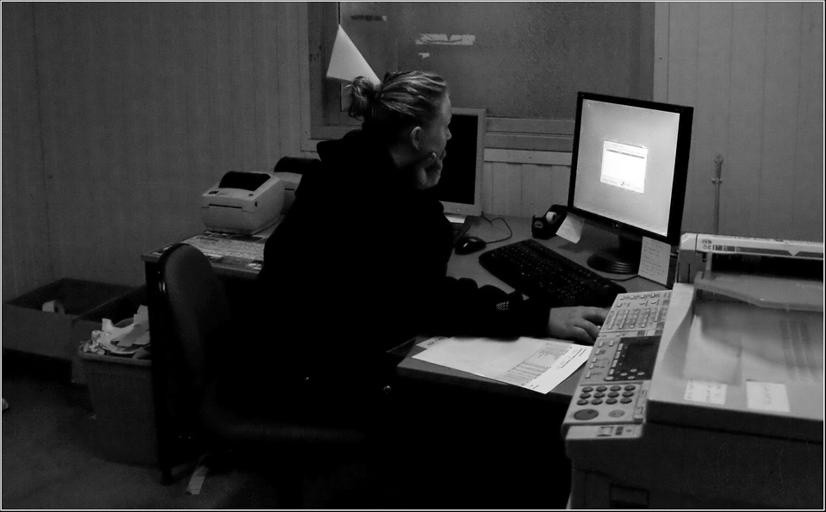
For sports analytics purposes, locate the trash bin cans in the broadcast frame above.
[79,341,156,467]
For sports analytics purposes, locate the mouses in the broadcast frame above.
[456,237,484,256]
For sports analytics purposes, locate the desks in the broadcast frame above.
[142,214,674,404]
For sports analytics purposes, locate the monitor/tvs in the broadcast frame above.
[431,107,486,216]
[568,90,694,273]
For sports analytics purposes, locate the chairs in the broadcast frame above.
[147,242,376,508]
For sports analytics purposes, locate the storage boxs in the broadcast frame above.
[2,276,135,405]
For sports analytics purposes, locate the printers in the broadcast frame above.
[559,232,822,509]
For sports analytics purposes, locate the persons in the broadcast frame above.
[259,65,612,506]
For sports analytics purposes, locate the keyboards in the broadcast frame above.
[480,238,627,309]
[180,235,266,263]
